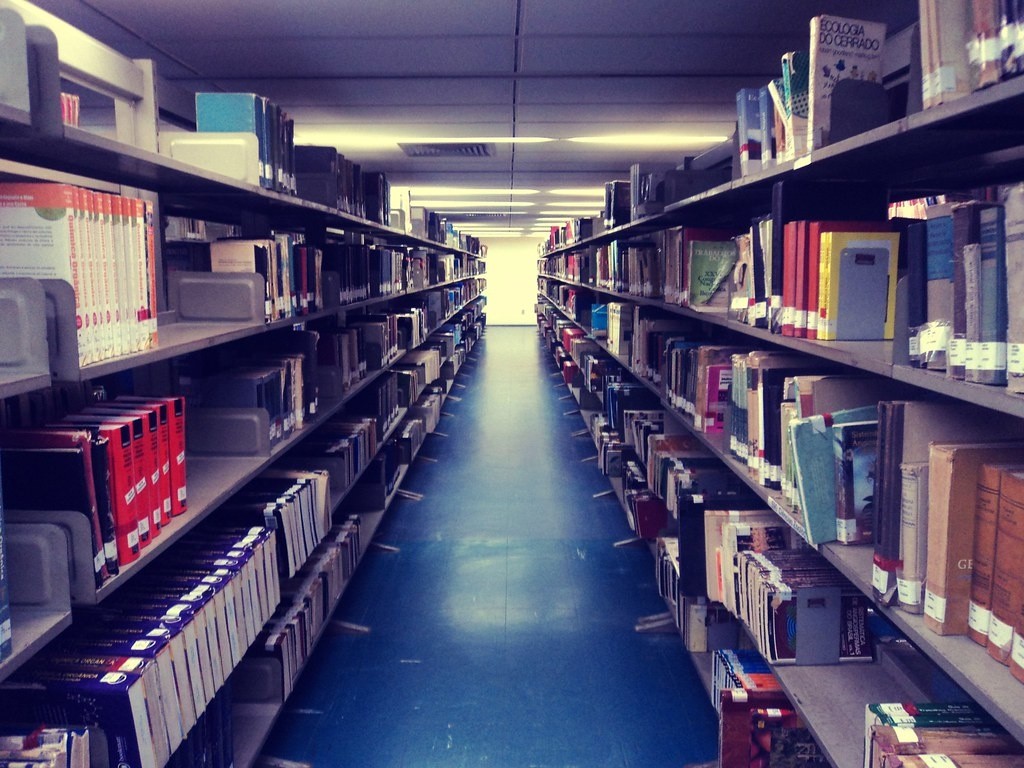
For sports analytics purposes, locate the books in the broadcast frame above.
[711,648,828,767]
[232,514,362,700]
[0,89,159,366]
[195,92,297,194]
[0,459,281,768]
[537,1,1023,690]
[204,468,333,578]
[182,329,320,449]
[290,144,487,509]
[864,700,1022,767]
[1,395,187,587]
[164,204,324,324]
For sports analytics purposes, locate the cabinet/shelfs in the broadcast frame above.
[537,75,1024,768]
[0,102,489,768]
